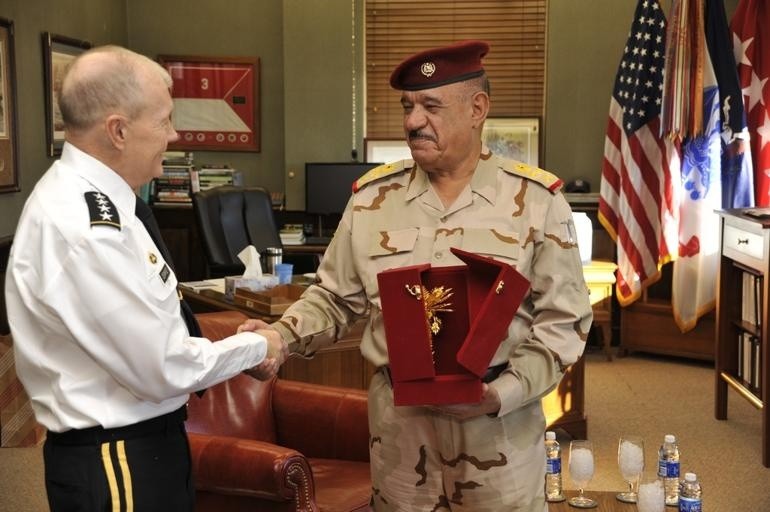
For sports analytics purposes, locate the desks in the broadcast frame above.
[283,244,331,256]
[178,274,380,395]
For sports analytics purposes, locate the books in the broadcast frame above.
[153,151,235,206]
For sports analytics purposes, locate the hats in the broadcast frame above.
[388,41,491,92]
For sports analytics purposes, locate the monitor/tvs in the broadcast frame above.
[306,161,385,216]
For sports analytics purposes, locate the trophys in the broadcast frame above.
[422,284,454,364]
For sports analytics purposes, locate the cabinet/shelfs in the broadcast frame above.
[147,170,243,279]
[570,197,621,362]
[714,208,770,472]
[616,282,717,366]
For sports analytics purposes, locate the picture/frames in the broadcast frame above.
[154,54,263,155]
[362,135,414,167]
[480,114,543,172]
[42,29,99,159]
[0,14,23,195]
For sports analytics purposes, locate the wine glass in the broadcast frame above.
[635,480,667,512]
[568,438,598,507]
[617,436,645,503]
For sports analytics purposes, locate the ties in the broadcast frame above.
[135,195,207,400]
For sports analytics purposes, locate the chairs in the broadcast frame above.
[190,186,325,275]
[181,307,376,512]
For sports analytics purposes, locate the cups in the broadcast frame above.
[274,263,294,284]
[266,247,283,275]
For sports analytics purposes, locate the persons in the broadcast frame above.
[240,43,595,512]
[5,44,288,512]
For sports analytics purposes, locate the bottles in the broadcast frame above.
[544,431,562,500]
[657,433,680,505]
[678,472,702,512]
